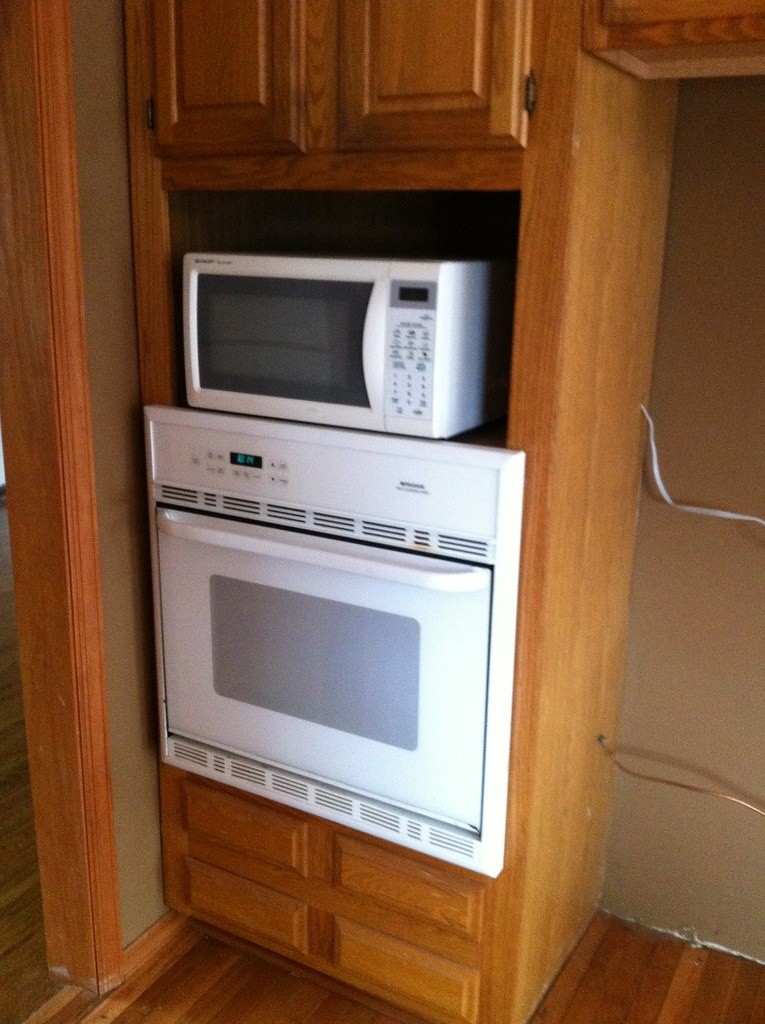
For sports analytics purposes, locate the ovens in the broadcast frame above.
[136,402,529,878]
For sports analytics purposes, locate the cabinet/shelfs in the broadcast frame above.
[122,2,679,1024]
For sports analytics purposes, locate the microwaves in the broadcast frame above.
[183,250,517,440]
